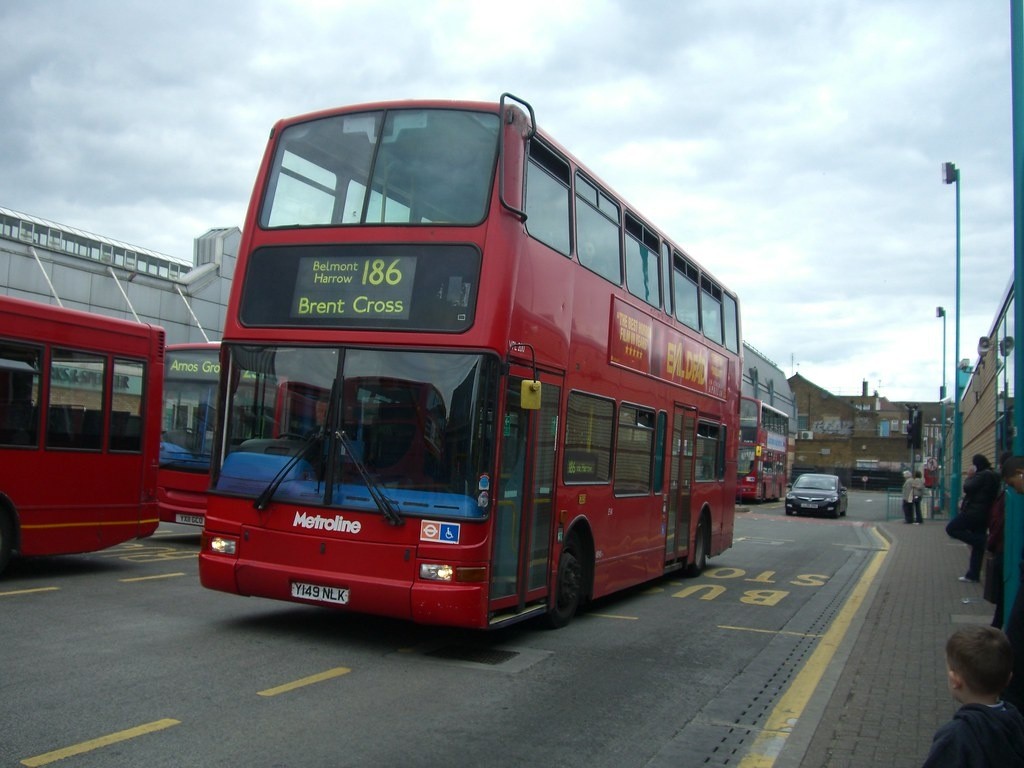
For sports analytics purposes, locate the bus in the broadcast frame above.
[197,93,745,631]
[738,396,789,502]
[154,342,333,525]
[0,294,166,582]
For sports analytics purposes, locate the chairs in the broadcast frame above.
[0,397,141,451]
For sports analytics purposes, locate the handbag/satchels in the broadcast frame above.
[912,496,921,503]
[908,488,913,503]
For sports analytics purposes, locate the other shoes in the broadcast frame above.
[958,577,975,582]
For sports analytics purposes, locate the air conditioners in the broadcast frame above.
[802,430,814,439]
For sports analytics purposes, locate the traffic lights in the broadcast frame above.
[906,423,915,443]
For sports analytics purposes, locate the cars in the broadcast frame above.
[786,474,848,518]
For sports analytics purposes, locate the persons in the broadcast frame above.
[945,454,999,583]
[912,471,925,525]
[1001,456,1024,716]
[300,396,333,452]
[983,453,1013,630]
[902,471,914,524]
[922,625,1024,768]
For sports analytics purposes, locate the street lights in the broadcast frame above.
[937,306,946,508]
[941,161,963,520]
